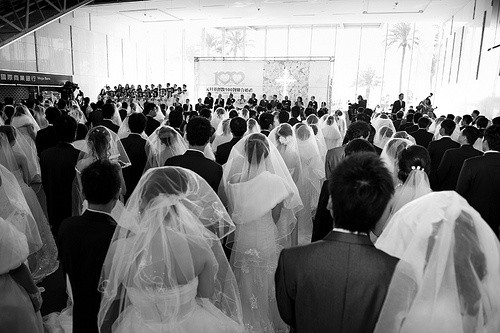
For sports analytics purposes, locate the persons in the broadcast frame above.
[370,144,434,244]
[373,189,499,332]
[40,114,84,239]
[0,165,45,279]
[218,132,305,332]
[456,128,500,240]
[43,124,140,332]
[0,177,45,333]
[0,78,499,218]
[55,158,142,332]
[266,122,313,249]
[96,164,249,333]
[311,138,376,243]
[273,150,420,332]
[1,123,61,285]
[290,121,326,245]
[164,115,226,232]
